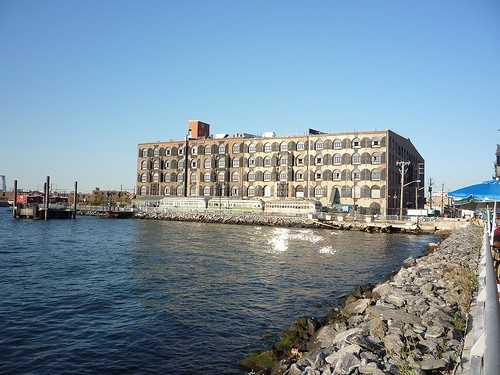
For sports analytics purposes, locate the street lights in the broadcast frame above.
[397,160,422,220]
